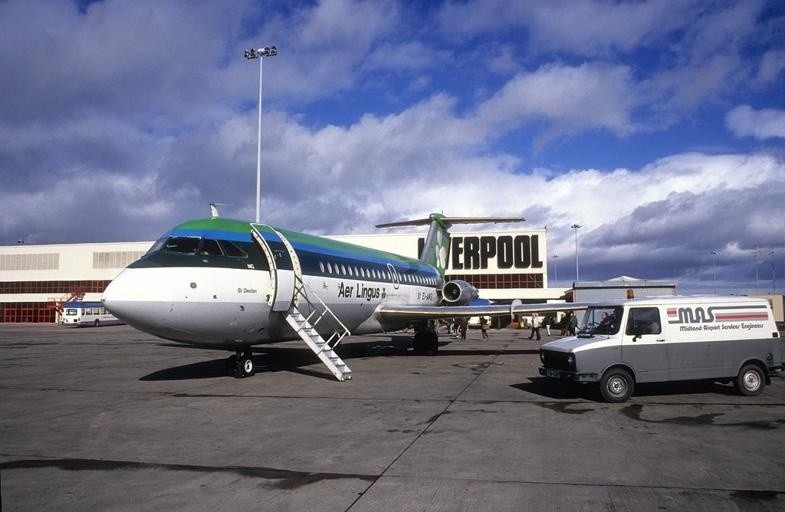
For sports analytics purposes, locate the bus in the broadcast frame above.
[59,300,127,328]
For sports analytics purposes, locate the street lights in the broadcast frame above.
[711,249,720,295]
[244,44,279,225]
[769,251,777,292]
[552,254,559,287]
[752,246,761,286]
[571,223,581,281]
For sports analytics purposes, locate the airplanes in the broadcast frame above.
[98,202,591,382]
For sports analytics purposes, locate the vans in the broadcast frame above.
[538,292,783,402]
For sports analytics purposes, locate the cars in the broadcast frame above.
[526,318,545,328]
[468,316,491,329]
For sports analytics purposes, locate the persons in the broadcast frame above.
[479,315,489,341]
[441,316,470,340]
[599,312,611,329]
[544,313,552,336]
[557,312,578,336]
[528,313,541,340]
[641,310,659,334]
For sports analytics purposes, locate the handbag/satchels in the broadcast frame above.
[482,323,489,331]
